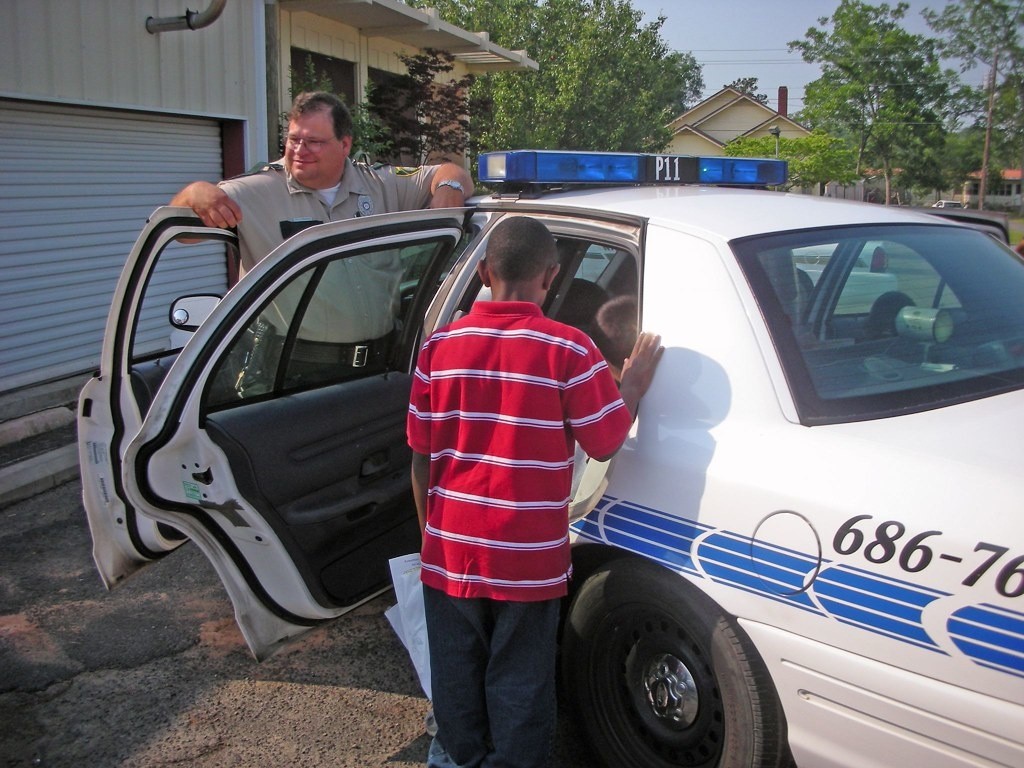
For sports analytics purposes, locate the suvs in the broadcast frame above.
[73,148,1023,767]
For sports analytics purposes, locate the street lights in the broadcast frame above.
[767,125,781,191]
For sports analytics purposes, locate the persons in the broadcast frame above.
[167,91,475,403]
[404,216,665,768]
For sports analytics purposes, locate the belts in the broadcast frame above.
[270,340,392,368]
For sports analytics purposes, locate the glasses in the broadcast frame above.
[282,135,338,152]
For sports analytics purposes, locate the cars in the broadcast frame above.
[931,200,963,210]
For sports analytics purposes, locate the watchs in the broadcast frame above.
[437,180,465,195]
